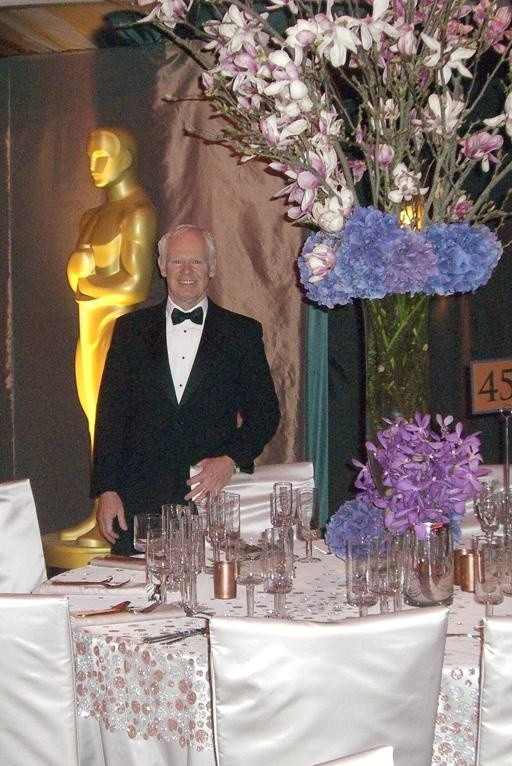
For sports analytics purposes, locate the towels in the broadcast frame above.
[41,582,154,595]
[72,604,190,628]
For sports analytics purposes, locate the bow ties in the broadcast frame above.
[171,307,204,325]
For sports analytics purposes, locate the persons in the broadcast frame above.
[91,225,280,557]
[58,127,157,548]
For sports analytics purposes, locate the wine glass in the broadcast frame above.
[346,479,512,618]
[124,481,321,621]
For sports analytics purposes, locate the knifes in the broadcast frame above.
[144,627,207,644]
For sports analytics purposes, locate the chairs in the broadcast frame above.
[201,602,454,766]
[0,591,80,766]
[0,476,51,593]
[475,616,510,764]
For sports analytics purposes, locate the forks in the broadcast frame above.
[125,601,162,613]
[53,579,131,588]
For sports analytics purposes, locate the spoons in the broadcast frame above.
[78,601,130,617]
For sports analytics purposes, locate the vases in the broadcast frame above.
[353,282,439,454]
[400,520,456,609]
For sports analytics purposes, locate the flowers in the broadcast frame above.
[350,408,495,597]
[120,0,512,441]
